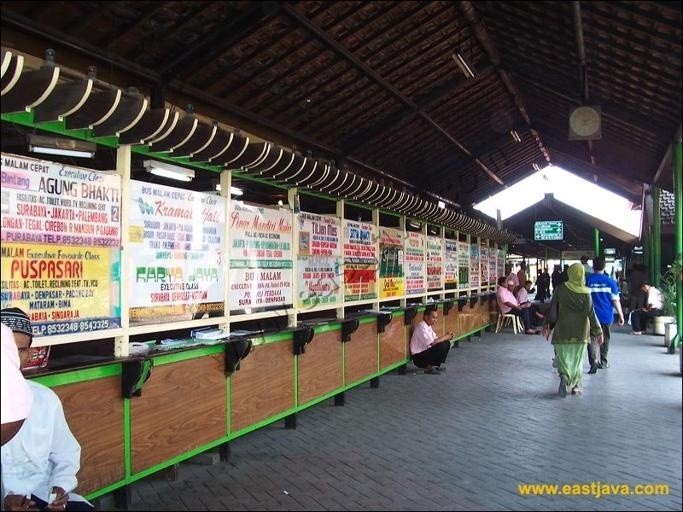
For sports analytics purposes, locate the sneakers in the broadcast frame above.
[572,387,582,395]
[558,376,568,398]
[525,330,540,335]
[600,364,610,369]
[629,330,646,335]
[587,360,599,374]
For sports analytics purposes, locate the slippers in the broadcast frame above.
[424,366,446,375]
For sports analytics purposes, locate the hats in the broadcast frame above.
[508,280,514,285]
[1,307,32,336]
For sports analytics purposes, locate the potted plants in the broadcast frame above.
[654,262,677,346]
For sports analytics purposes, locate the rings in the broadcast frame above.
[63,504,66,511]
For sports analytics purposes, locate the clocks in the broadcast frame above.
[567,103,602,141]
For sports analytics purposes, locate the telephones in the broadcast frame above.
[129,342,149,354]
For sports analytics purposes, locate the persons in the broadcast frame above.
[410,308,454,375]
[496,255,663,398]
[0,308,95,511]
[0,322,31,444]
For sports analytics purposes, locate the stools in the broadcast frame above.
[653,315,676,336]
[495,311,525,335]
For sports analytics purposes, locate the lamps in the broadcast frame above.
[510,129,523,144]
[532,161,541,172]
[215,183,244,197]
[142,160,196,183]
[25,133,97,160]
[451,51,477,81]
